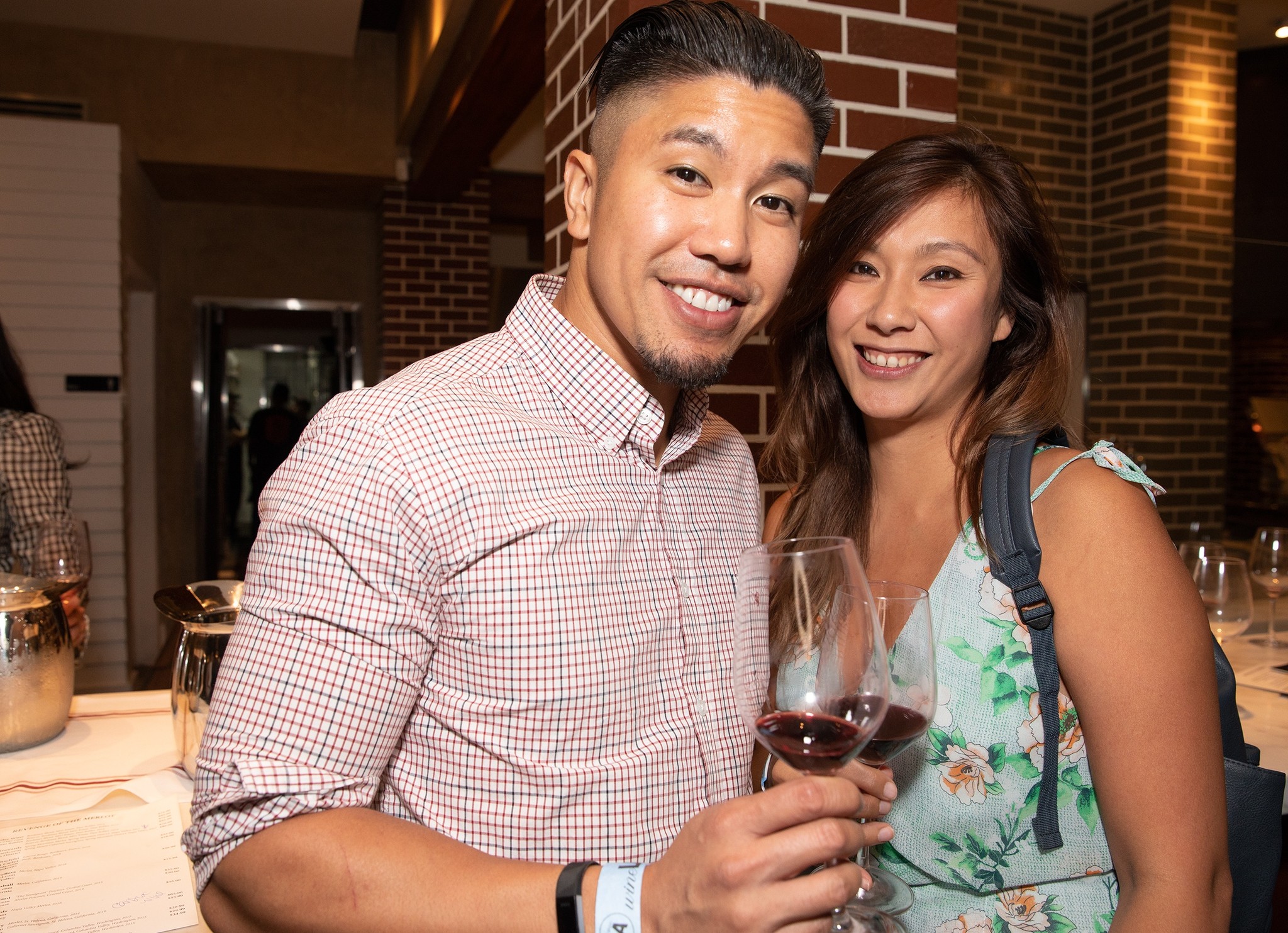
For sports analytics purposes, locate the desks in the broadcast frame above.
[0,684,216,933]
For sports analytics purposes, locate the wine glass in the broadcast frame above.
[1247,527,1288,648]
[736,537,938,933]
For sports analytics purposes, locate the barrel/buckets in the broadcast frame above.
[153,579,245,780]
[0,572,81,754]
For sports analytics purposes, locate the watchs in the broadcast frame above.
[554,857,599,932]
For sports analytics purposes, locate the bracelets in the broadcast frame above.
[598,861,644,932]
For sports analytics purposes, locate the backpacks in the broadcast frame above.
[981,423,1287,933]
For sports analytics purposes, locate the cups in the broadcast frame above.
[1179,540,1253,638]
[33,519,92,601]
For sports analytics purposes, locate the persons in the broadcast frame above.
[177,0,907,933]
[245,380,302,536]
[778,134,1234,932]
[225,391,249,532]
[287,396,307,420]
[1,322,82,582]
